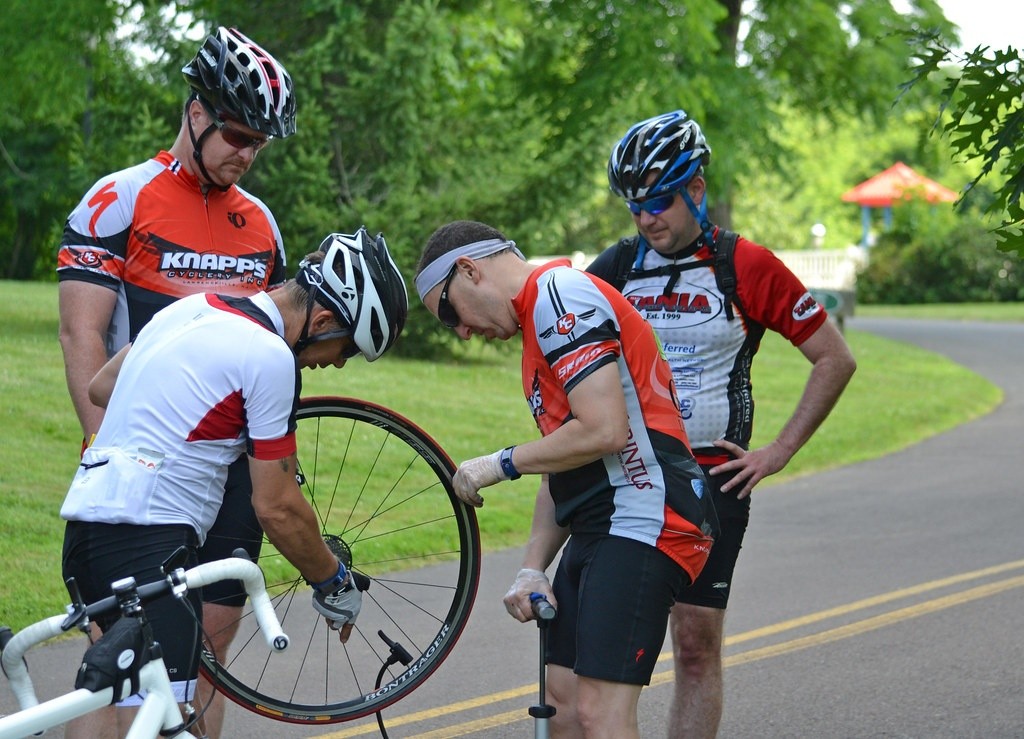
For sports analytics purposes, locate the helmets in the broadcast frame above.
[183,27,296,137]
[608,110,711,201]
[292,226,408,362]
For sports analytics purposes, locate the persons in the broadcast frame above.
[59,223,409,739]
[55,25,296,739]
[414,220,722,739]
[583,109,857,739]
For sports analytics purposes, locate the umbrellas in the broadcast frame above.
[843,163,958,207]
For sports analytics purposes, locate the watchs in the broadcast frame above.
[501,445,522,481]
[303,559,347,595]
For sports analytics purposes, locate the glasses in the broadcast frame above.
[204,107,273,151]
[438,263,461,328]
[626,188,685,216]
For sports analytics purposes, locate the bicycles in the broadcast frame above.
[0,394,481,739]
